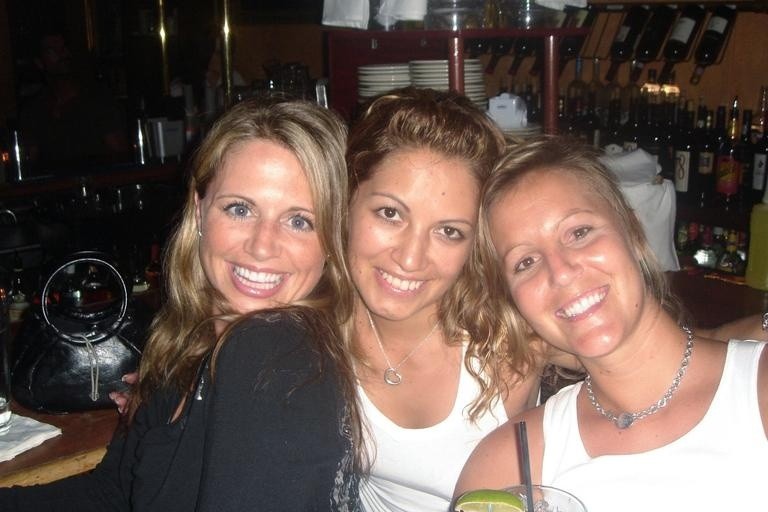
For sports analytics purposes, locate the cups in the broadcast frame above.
[500,485,585,511]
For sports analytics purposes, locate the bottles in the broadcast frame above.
[490,1,767,287]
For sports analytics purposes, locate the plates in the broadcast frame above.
[357,57,488,110]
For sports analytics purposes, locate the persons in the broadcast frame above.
[0,87,371,512]
[337,83,544,512]
[441,131,768,511]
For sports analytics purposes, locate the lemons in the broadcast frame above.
[454,489,527,512]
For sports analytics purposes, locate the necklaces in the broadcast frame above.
[365,309,444,385]
[583,325,697,431]
